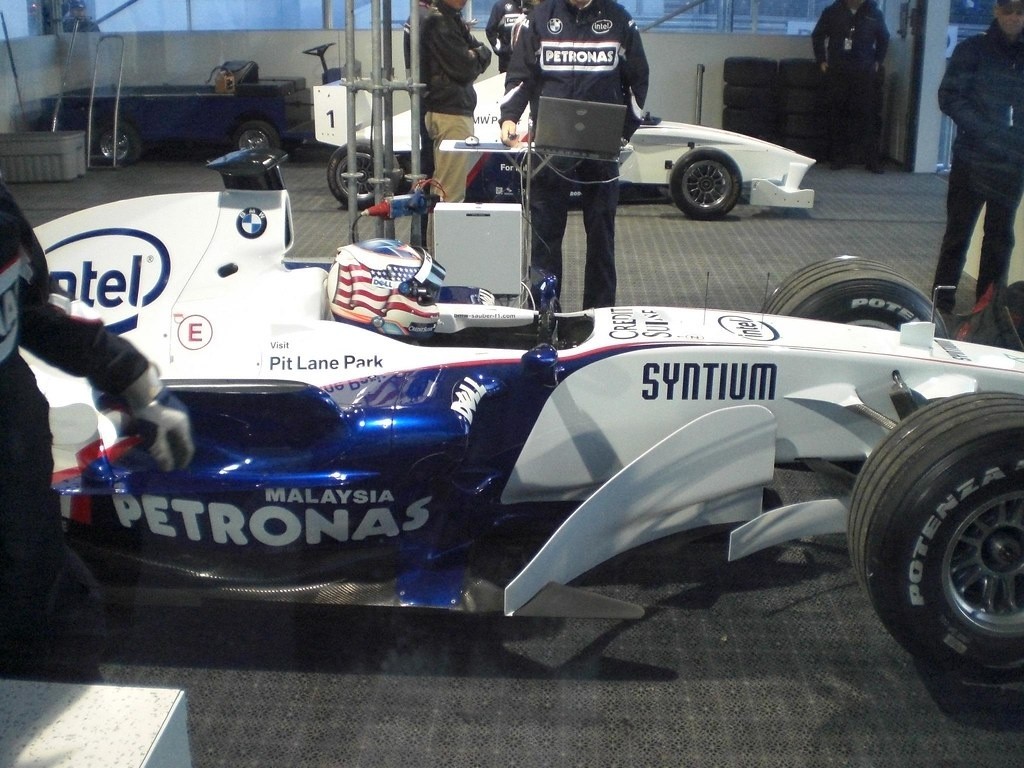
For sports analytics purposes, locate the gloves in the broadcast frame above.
[120,362,195,472]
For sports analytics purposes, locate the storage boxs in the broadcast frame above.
[0,131,87,185]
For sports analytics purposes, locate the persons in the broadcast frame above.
[42,0,99,34]
[403,1,649,315]
[326,238,447,345]
[0,183,194,684]
[812,0,891,174]
[932,0,1024,323]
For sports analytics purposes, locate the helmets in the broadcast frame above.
[327,238,446,339]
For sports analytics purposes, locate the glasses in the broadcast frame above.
[1000,5,1024,15]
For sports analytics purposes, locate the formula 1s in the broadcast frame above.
[22,188,1024,693]
[314,68,819,222]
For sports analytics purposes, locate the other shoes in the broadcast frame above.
[866,165,884,174]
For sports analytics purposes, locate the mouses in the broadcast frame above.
[465,135,479,146]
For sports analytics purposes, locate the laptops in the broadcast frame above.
[534,96,627,154]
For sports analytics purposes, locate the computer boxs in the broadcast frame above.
[434,202,522,296]
